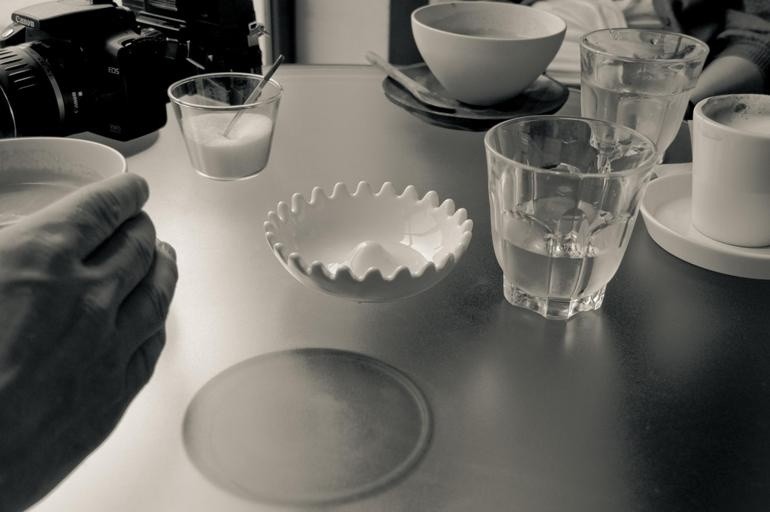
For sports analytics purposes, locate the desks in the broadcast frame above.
[31,62,768,510]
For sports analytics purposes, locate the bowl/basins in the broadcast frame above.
[413,0,568,104]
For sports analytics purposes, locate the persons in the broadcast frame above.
[431,0,769,121]
[0,173,181,512]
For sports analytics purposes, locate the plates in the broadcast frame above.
[382,64,571,131]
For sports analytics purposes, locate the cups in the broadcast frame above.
[166,70,282,181]
[577,26,710,165]
[0,133,127,231]
[483,114,660,319]
[691,94,770,251]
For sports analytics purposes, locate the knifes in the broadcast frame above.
[363,46,454,112]
